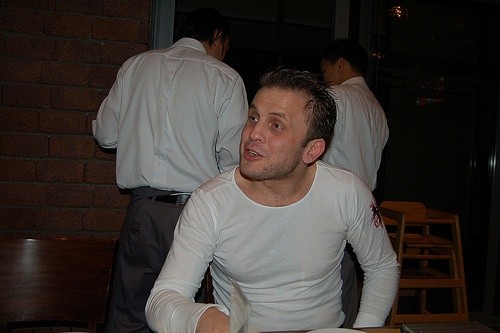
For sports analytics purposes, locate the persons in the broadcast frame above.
[144,66,401,333]
[92,8,249,333]
[318,37,389,332]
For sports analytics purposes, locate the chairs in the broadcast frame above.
[0,232,119,333]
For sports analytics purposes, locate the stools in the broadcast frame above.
[381,200,469,327]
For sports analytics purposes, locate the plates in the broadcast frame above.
[306,328,367,333]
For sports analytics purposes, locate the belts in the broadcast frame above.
[148,194,191,205]
